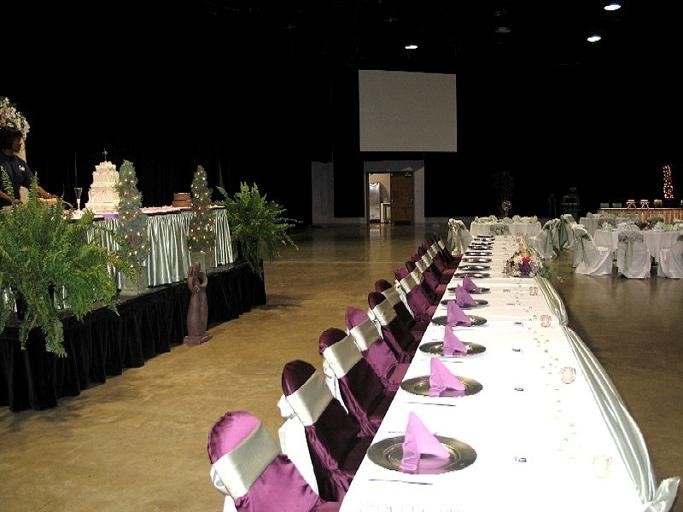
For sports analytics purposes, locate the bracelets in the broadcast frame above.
[45,193,50,198]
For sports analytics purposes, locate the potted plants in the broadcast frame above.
[0,164,143,357]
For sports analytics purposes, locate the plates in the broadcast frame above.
[367,433,475,474]
[431,315,488,327]
[441,235,495,307]
[418,340,486,357]
[400,375,482,397]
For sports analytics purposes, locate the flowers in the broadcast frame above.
[0,94,33,145]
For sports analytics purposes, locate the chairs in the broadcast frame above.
[446,198,682,280]
[274,359,374,503]
[319,325,397,438]
[208,409,343,511]
[343,223,464,393]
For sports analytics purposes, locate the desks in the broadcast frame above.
[4,206,235,314]
[340,279,682,511]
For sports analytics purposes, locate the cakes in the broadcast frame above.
[85,160,120,213]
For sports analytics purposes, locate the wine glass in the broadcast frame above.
[73,187,82,209]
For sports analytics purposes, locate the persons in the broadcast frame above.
[0,126,58,211]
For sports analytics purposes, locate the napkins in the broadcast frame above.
[400,410,449,473]
[455,284,478,307]
[446,300,474,326]
[426,356,467,395]
[461,275,479,292]
[443,322,467,358]
